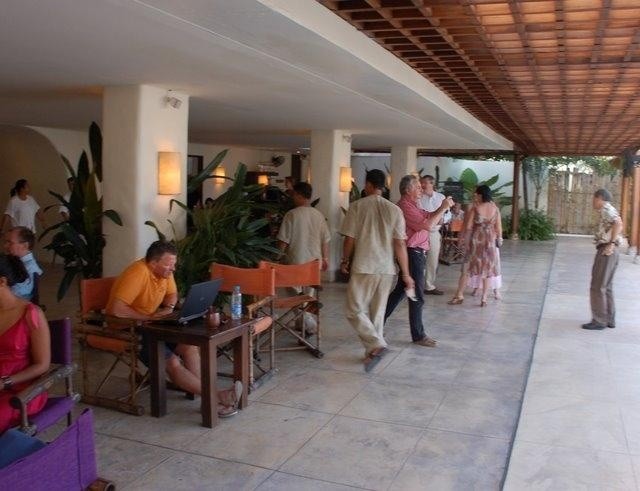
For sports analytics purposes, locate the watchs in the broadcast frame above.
[164,304,175,309]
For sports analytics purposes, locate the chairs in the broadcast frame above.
[207,261,277,397]
[13,315,82,440]
[2,407,117,491]
[76,277,183,417]
[254,258,325,360]
[441,220,467,266]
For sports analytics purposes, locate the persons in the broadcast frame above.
[60,176,88,268]
[3,225,44,303]
[0,253,51,437]
[276,181,331,336]
[105,240,243,418]
[0,178,55,257]
[188,175,299,238]
[339,169,503,373]
[582,188,622,330]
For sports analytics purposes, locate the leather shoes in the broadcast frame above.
[423,288,443,295]
[583,323,603,330]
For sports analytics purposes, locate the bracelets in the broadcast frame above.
[0,376,12,391]
[610,241,615,244]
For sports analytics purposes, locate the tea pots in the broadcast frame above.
[203,305,226,331]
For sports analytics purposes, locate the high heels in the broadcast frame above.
[481,297,486,306]
[447,296,464,305]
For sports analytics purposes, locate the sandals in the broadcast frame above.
[219,381,243,418]
[364,347,385,372]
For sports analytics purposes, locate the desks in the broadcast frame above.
[139,315,258,430]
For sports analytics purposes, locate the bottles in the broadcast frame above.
[230,286,242,320]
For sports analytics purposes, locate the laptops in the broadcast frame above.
[152,280,224,325]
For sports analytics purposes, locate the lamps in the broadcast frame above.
[216,167,227,185]
[340,166,356,194]
[157,152,182,196]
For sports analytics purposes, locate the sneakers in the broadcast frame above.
[413,335,438,347]
